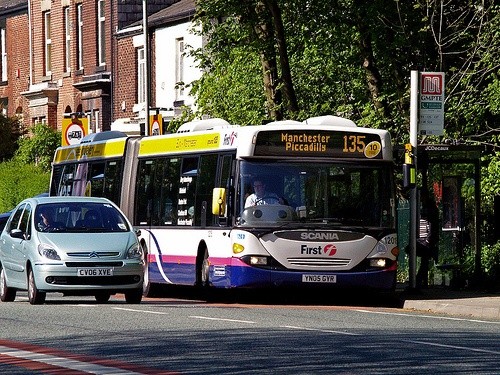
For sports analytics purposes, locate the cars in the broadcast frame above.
[0,192,49,233]
[0,196,146,305]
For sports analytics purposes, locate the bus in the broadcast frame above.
[45,115,410,304]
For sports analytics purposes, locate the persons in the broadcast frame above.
[37,207,62,232]
[243,177,284,211]
